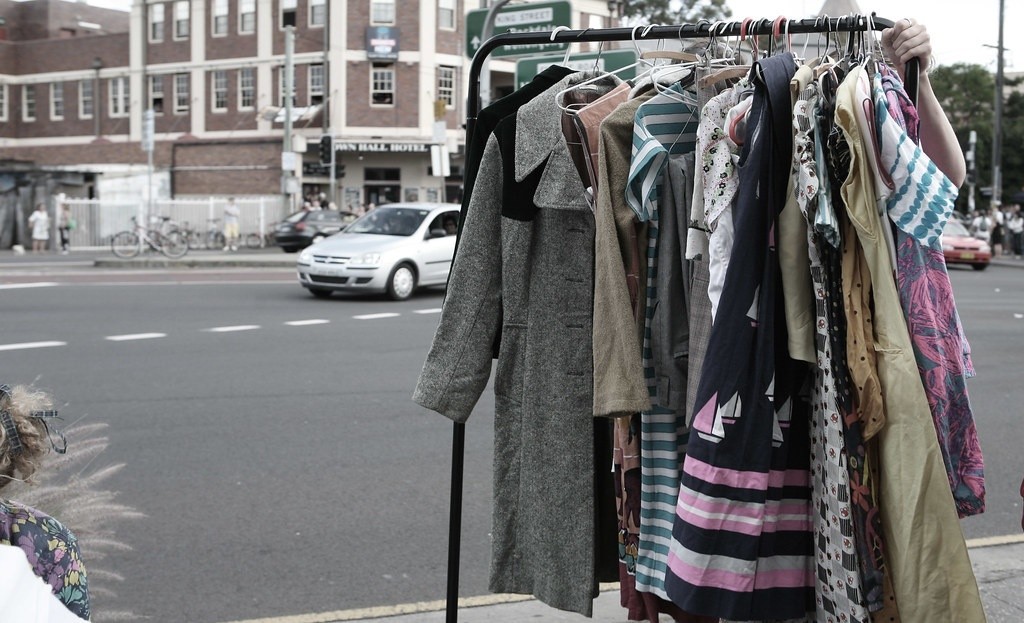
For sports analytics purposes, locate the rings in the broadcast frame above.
[904,17,913,26]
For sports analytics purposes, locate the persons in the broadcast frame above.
[965,197,1023,259]
[221,196,240,252]
[295,185,384,229]
[670,16,967,192]
[57,202,71,256]
[28,201,52,254]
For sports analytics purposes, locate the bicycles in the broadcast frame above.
[111,213,264,258]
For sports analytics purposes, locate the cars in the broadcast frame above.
[267,210,361,253]
[297,203,465,303]
[938,215,991,272]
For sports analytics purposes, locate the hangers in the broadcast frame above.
[549,12,893,158]
